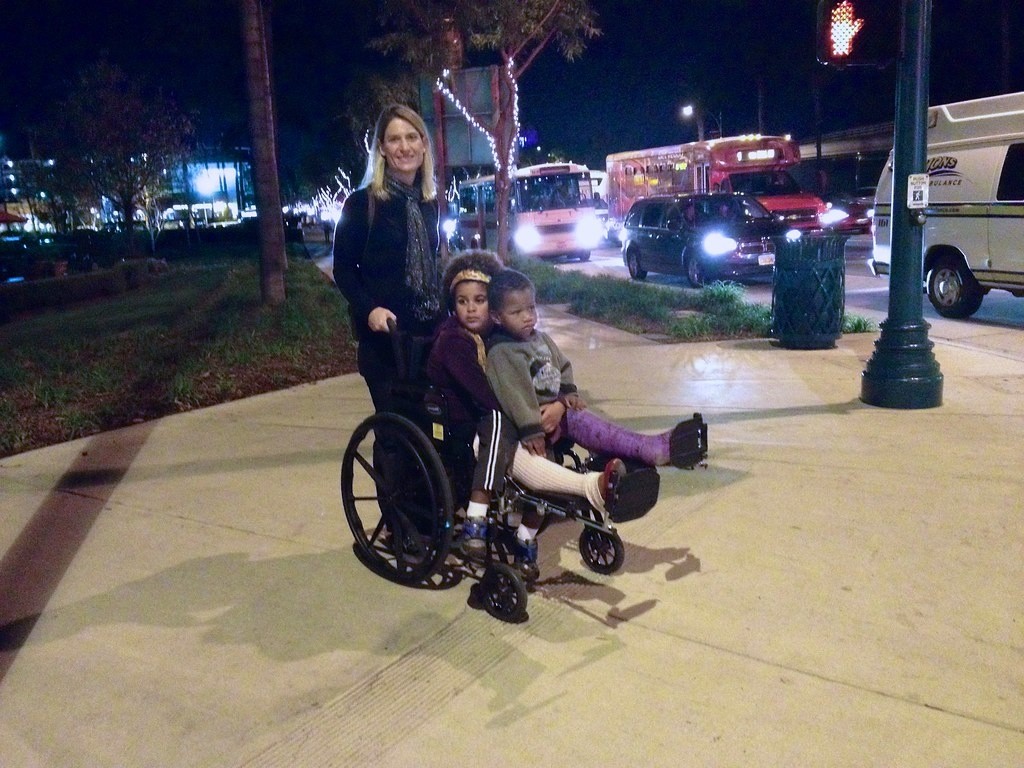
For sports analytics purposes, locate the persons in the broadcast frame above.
[464,268,586,563]
[332,104,451,545]
[425,251,675,515]
[593,192,608,224]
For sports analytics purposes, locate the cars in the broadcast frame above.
[845,186,876,211]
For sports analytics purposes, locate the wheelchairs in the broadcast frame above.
[339,314,625,625]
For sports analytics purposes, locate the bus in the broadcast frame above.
[605,132,833,246]
[446,161,606,263]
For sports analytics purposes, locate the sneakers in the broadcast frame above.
[461,514,489,563]
[513,529,540,581]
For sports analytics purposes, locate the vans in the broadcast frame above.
[870,91,1024,320]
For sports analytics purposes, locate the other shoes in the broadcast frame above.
[598,458,627,503]
[384,524,433,564]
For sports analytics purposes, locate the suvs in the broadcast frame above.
[619,190,801,285]
